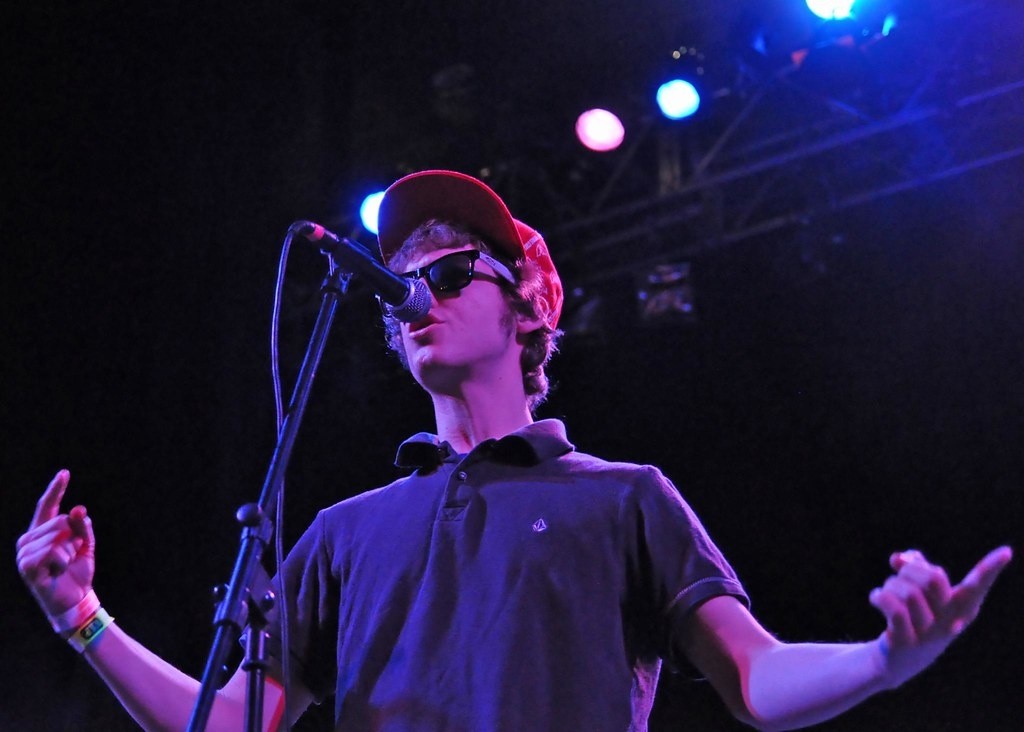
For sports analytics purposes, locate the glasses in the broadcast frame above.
[379,249,520,318]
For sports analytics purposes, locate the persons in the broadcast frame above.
[17,170,1012,732]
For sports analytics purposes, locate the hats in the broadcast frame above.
[377,170,565,331]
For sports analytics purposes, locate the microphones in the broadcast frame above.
[297,222,433,323]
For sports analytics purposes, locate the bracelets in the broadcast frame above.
[48,589,101,633]
[67,608,115,654]
